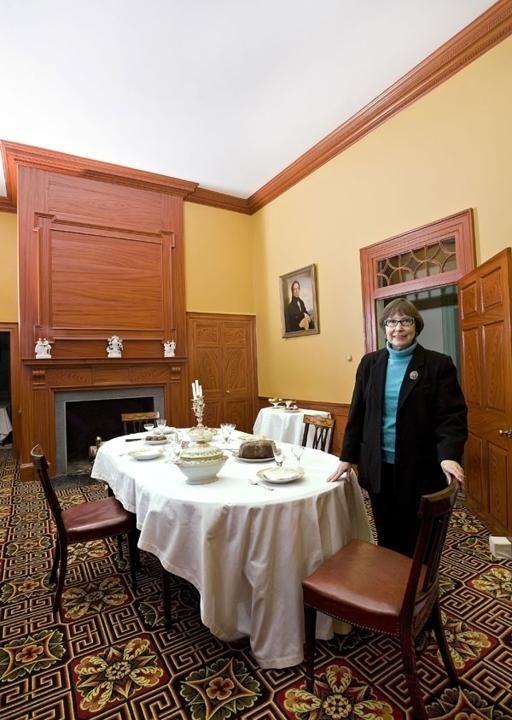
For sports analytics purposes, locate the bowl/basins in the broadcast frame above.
[175,456,229,485]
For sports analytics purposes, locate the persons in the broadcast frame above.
[34,338,44,355]
[163,340,171,358]
[324,300,468,656]
[42,337,49,355]
[169,339,176,357]
[284,280,315,331]
[106,335,123,359]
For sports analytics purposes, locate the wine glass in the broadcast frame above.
[156,420,166,436]
[144,424,154,435]
[291,446,304,469]
[273,444,286,470]
[220,423,236,449]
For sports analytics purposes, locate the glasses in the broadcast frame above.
[384,320,413,326]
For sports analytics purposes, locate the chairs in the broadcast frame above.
[302,415,336,454]
[30,443,137,613]
[121,411,160,435]
[302,473,460,720]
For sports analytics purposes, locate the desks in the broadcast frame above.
[0,400,13,446]
[253,405,333,453]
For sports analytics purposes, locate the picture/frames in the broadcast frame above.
[279,264,320,338]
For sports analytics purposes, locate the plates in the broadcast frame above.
[128,450,162,460]
[258,465,304,484]
[143,437,168,445]
[234,453,276,463]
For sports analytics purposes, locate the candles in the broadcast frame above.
[192,380,203,400]
